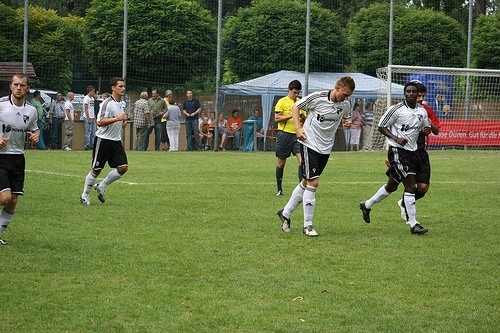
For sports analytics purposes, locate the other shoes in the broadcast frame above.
[65,147,73,150]
[0,238,8,245]
[277,190,284,196]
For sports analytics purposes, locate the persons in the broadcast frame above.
[133,92,151,151]
[30,91,46,150]
[182,90,202,153]
[0,72,41,245]
[102,93,109,100]
[441,105,455,150]
[276,76,355,238]
[274,79,306,196]
[80,76,129,206]
[147,88,168,151]
[359,82,431,234]
[350,101,374,151]
[199,109,242,151]
[385,82,442,221]
[48,91,65,150]
[82,85,96,150]
[161,89,182,153]
[248,109,264,139]
[64,91,75,151]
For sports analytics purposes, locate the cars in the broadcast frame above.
[28,90,105,119]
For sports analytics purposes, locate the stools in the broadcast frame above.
[269,135,276,151]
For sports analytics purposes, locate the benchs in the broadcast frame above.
[191,133,269,151]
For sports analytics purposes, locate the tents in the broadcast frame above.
[218,70,407,152]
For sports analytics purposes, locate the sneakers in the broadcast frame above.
[80,197,92,206]
[359,201,371,223]
[410,223,428,234]
[93,183,105,203]
[398,199,407,220]
[277,208,291,232]
[303,225,318,236]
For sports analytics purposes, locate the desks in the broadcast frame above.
[266,128,279,151]
